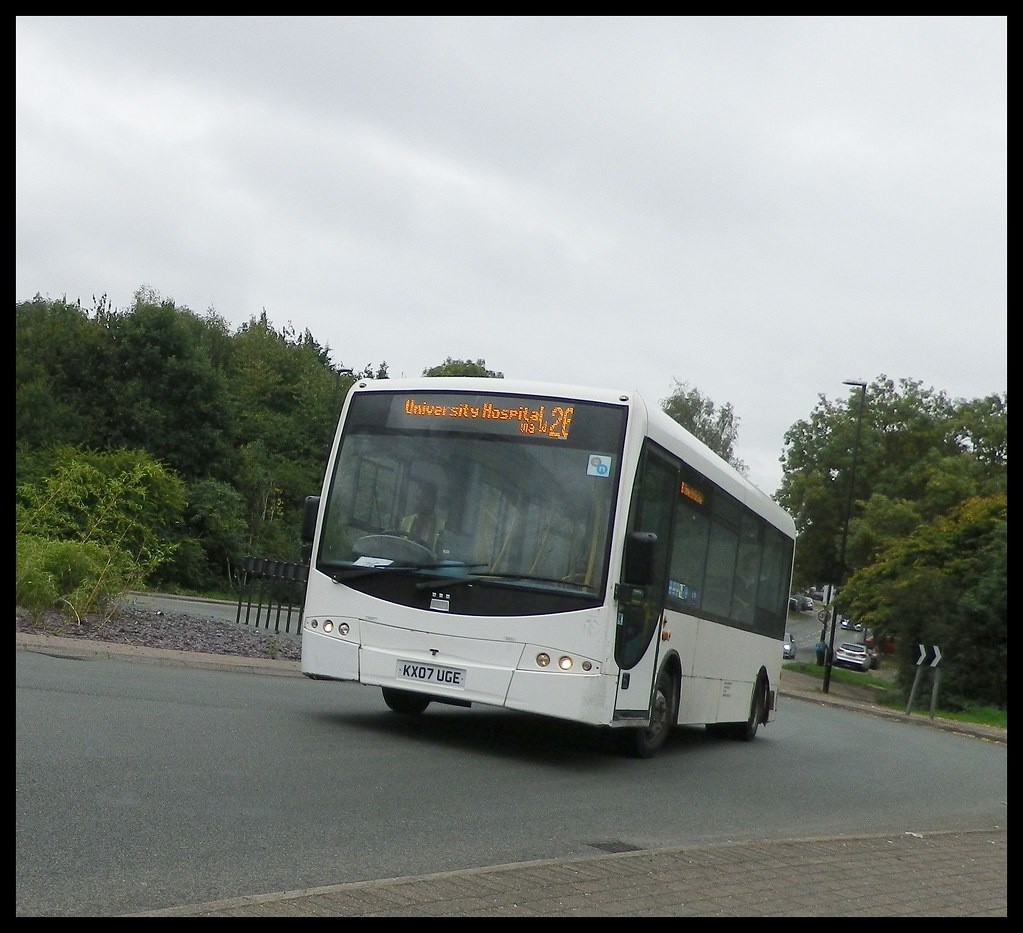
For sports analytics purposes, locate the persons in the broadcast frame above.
[383,481,450,557]
[619,550,766,672]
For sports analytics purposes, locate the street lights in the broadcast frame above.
[821,376,868,694]
[326,368,352,460]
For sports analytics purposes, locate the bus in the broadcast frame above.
[299,379,797,758]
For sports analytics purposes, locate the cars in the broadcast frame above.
[782,632,796,660]
[789,595,814,612]
[865,635,900,654]
[835,642,868,669]
[841,619,863,631]
